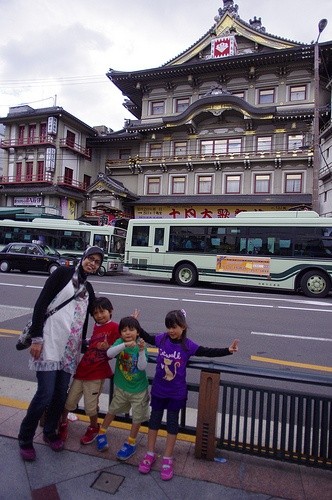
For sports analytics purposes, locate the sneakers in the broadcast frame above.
[42,434,64,451]
[80,422,101,444]
[116,442,137,460]
[21,446,35,459]
[139,453,156,474]
[160,457,175,480]
[57,421,68,441]
[97,434,108,451]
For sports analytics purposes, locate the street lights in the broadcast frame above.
[312,19,328,216]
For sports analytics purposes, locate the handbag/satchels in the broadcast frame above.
[15,321,33,350]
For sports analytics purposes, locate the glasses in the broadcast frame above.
[87,255,102,266]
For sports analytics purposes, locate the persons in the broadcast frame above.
[132,233,332,257]
[131,308,240,480]
[17,246,104,462]
[57,297,119,445]
[52,237,89,250]
[96,317,151,461]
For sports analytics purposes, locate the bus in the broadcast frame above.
[123,211,332,298]
[0,217,129,277]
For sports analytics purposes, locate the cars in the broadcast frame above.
[0,243,77,276]
